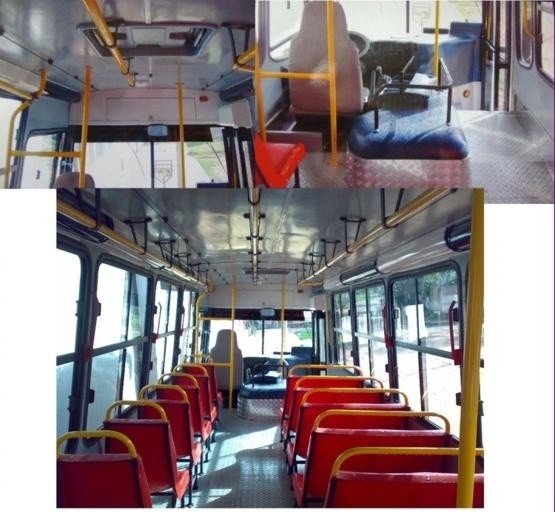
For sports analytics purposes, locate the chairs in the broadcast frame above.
[209,328,245,391]
[254,129,306,189]
[51,171,95,188]
[58,352,222,508]
[286,1,370,135]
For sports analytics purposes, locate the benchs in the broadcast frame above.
[277,361,485,507]
